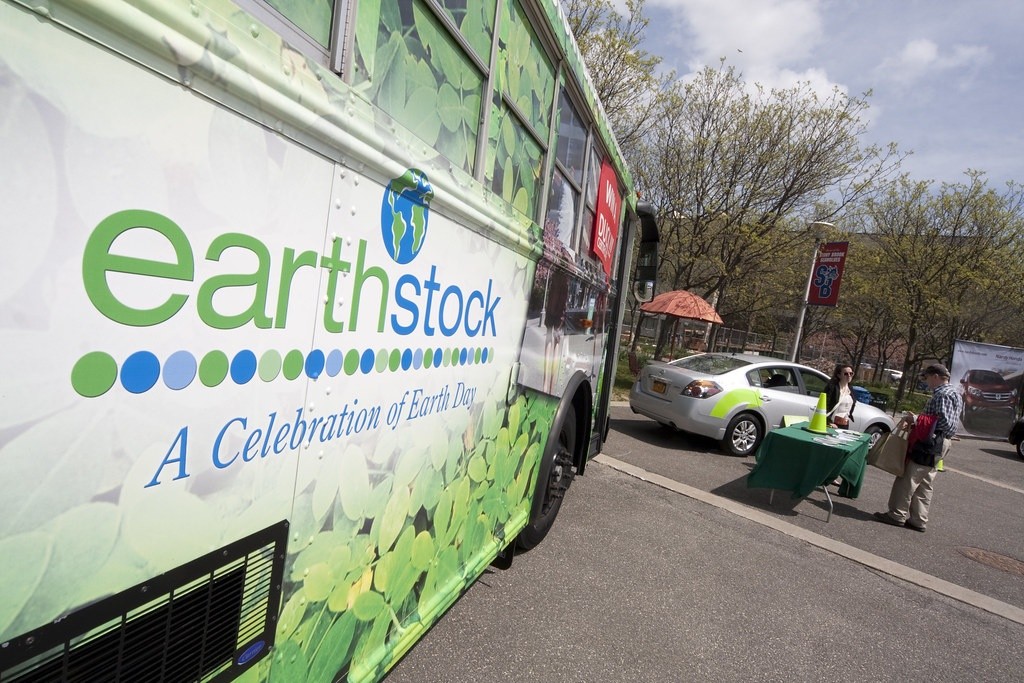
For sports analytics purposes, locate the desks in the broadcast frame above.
[747,421,871,524]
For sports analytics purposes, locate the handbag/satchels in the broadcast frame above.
[866,419,911,478]
[908,431,944,467]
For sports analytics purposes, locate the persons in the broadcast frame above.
[537,247,608,395]
[824,363,856,487]
[872,362,963,533]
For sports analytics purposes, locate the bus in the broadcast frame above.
[0,0,661,683]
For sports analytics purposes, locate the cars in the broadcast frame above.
[884,369,903,382]
[629,352,897,457]
[959,369,1017,428]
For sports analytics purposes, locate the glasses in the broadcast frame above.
[839,372,854,377]
[925,374,931,379]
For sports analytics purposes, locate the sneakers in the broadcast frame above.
[903,519,926,532]
[873,512,904,526]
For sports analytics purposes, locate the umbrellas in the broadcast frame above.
[638,288,725,362]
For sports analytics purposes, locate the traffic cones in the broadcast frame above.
[800,391,834,436]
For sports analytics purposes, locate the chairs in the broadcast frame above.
[784,415,809,428]
[764,374,787,391]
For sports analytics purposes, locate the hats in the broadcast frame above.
[920,364,950,377]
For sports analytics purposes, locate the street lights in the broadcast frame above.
[790,221,836,363]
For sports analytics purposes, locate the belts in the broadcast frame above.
[934,430,952,439]
